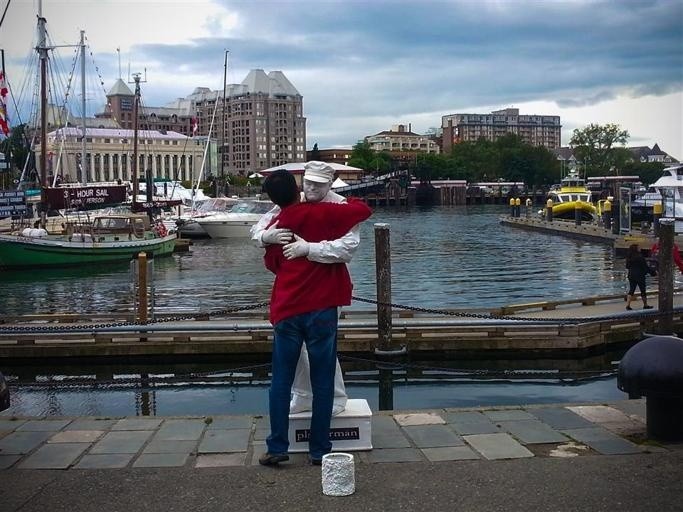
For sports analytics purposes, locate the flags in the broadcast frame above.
[0,70,10,138]
[192,116,198,136]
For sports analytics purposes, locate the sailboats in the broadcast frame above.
[0,0,188,269]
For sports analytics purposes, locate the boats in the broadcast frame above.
[541,154,599,221]
[648,165,682,234]
[169,190,274,244]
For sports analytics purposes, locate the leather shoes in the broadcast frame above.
[644,305,653,308]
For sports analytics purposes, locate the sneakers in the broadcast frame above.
[260,453,289,463]
[626,306,633,309]
[311,458,322,466]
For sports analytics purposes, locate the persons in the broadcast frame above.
[651,237,683,275]
[259,169,373,465]
[249,160,360,416]
[626,244,653,310]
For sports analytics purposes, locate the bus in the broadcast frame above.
[586,182,639,195]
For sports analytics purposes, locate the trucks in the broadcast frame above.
[636,181,646,192]
